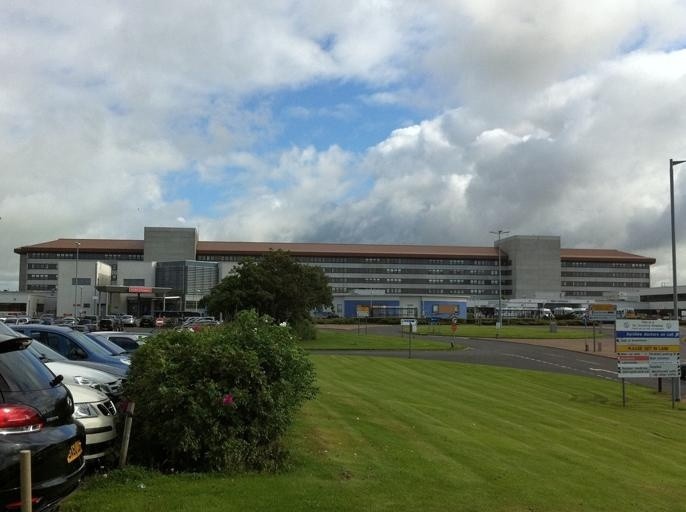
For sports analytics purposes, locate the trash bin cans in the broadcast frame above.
[401,319,417,332]
[551,322,557,331]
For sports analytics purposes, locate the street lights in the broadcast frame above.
[74,241,80,316]
[489,230,510,327]
[670,159,686,318]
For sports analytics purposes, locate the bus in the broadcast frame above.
[495,307,555,320]
[555,307,586,320]
